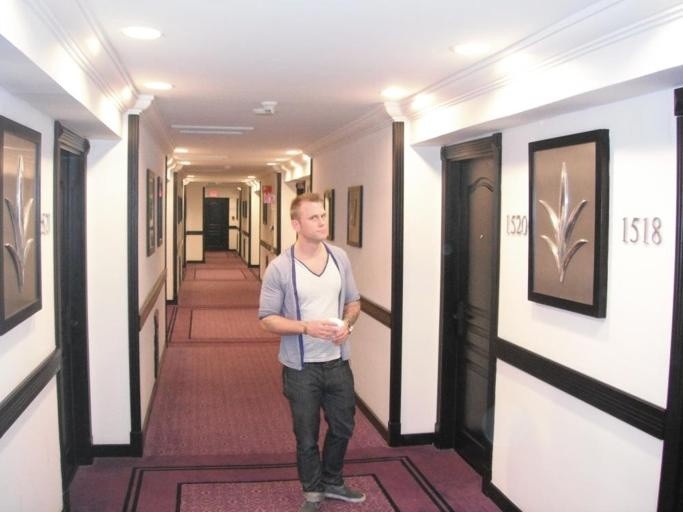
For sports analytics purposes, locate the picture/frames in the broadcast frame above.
[241,185,363,248]
[148,168,182,256]
[529,130,610,317]
[0,113,41,337]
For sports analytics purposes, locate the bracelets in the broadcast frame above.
[344,319,355,336]
[303,322,310,335]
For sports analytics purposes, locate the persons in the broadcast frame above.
[257,191,367,506]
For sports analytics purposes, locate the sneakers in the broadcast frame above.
[299,501,321,512]
[324,481,365,503]
